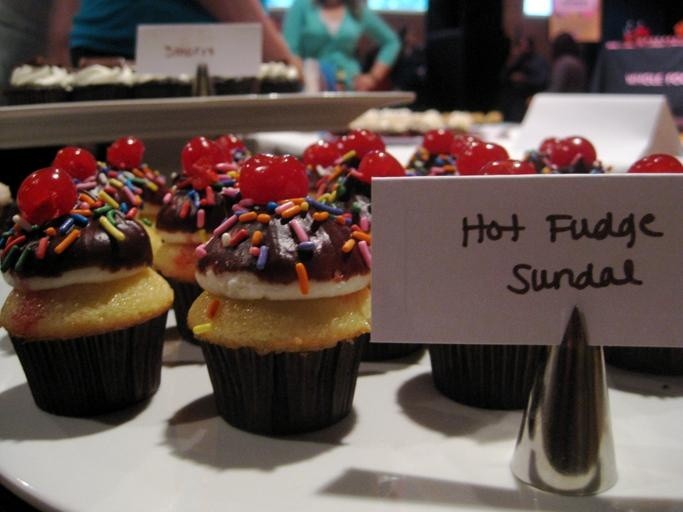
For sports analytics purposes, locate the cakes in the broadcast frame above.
[8,62,303,104]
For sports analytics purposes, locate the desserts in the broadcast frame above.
[0,127,683,435]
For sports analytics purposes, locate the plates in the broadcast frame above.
[1,90,417,149]
[0,269,681,510]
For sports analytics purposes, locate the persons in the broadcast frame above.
[46,1,682,135]
